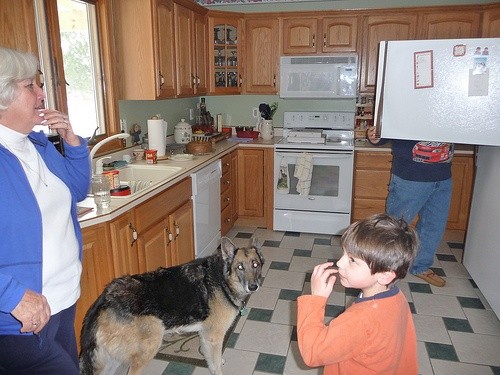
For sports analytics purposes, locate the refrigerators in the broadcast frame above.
[371,39,500,321]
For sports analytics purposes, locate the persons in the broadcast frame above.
[295,211,417,375]
[0,46,91,375]
[367,127,455,286]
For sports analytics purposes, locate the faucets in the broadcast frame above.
[90,132,131,177]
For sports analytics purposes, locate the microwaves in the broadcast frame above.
[280,53,359,100]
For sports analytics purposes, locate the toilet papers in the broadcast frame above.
[147,119,167,157]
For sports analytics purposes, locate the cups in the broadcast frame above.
[133,149,144,161]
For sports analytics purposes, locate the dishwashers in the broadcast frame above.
[190,159,222,262]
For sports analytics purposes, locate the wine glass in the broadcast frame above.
[214,27,240,89]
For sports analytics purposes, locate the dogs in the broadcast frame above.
[78,236,264,375]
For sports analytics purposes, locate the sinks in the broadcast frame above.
[119,166,186,184]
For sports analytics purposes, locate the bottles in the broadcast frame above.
[91,175,111,208]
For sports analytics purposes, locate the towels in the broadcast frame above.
[293,152,314,198]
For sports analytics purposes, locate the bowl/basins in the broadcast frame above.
[173,118,193,144]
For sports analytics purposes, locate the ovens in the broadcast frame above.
[273,143,355,236]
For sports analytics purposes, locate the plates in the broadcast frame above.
[168,153,194,162]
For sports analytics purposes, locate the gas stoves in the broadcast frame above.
[274,111,356,153]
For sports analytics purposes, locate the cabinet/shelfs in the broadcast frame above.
[112,0,500,103]
[218,147,273,236]
[76,176,195,358]
[352,153,475,245]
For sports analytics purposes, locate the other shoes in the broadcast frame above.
[409,268,446,287]
